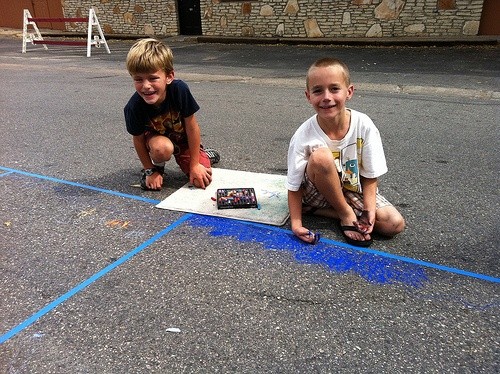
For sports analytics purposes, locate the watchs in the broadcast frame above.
[144,166,156,175]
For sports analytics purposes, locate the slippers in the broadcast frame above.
[303,203,319,216]
[338,219,373,246]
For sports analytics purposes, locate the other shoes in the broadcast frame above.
[204,148,220,165]
[141,164,164,191]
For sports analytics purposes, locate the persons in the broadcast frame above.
[286,57,404,246]
[123,38,220,189]
